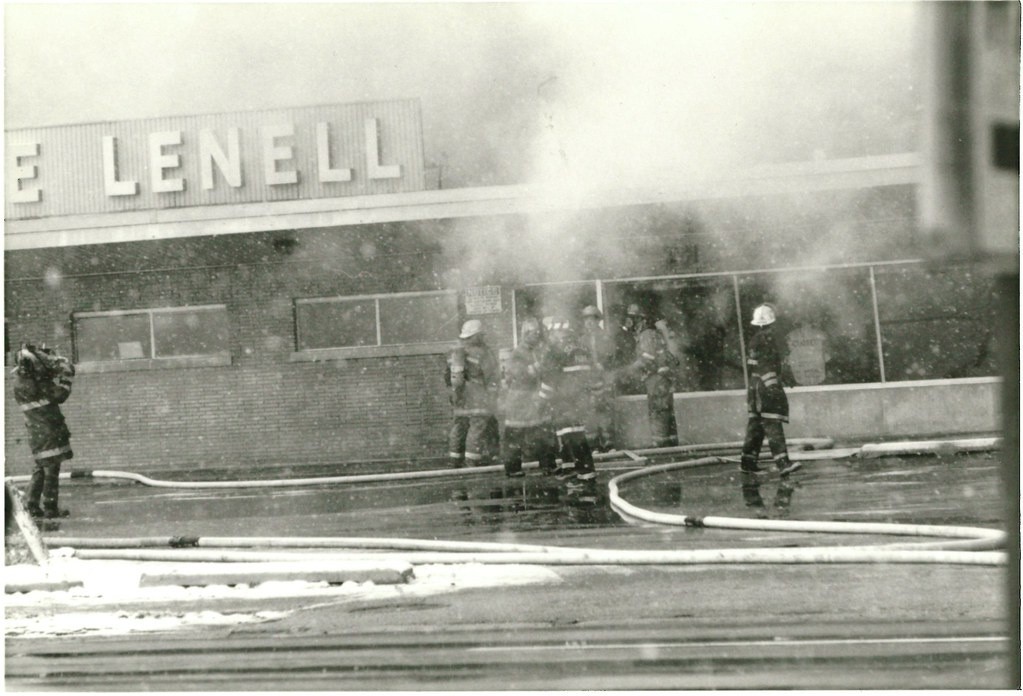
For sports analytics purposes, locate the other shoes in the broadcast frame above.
[27,502,44,517]
[742,475,769,487]
[578,490,599,507]
[777,455,802,477]
[565,477,596,488]
[504,469,525,477]
[540,455,557,469]
[554,466,577,480]
[740,460,768,474]
[447,461,462,469]
[43,501,70,518]
[779,479,802,492]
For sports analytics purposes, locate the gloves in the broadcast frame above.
[58,361,75,377]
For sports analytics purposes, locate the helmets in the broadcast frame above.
[751,305,776,326]
[626,303,650,319]
[459,319,483,338]
[580,305,604,319]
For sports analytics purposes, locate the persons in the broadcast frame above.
[10,344,74,518]
[446,302,678,488]
[739,304,803,476]
